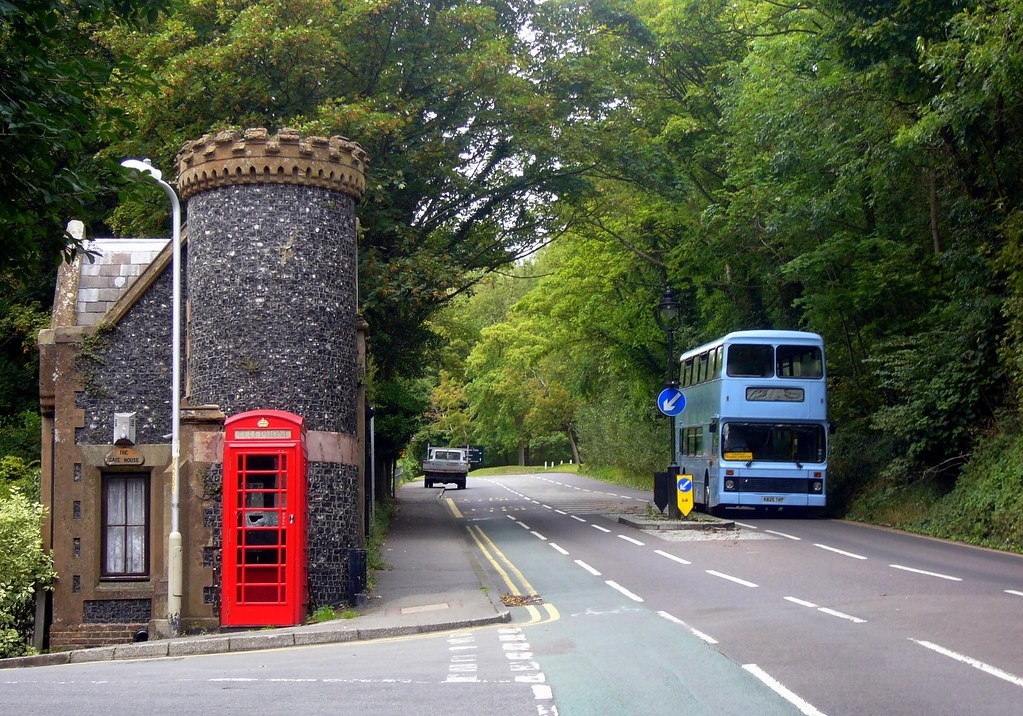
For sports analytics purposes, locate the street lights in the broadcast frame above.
[652,285,683,522]
[122,159,182,637]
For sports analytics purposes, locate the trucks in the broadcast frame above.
[422,444,468,490]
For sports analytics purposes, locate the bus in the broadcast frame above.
[675,330,838,516]
